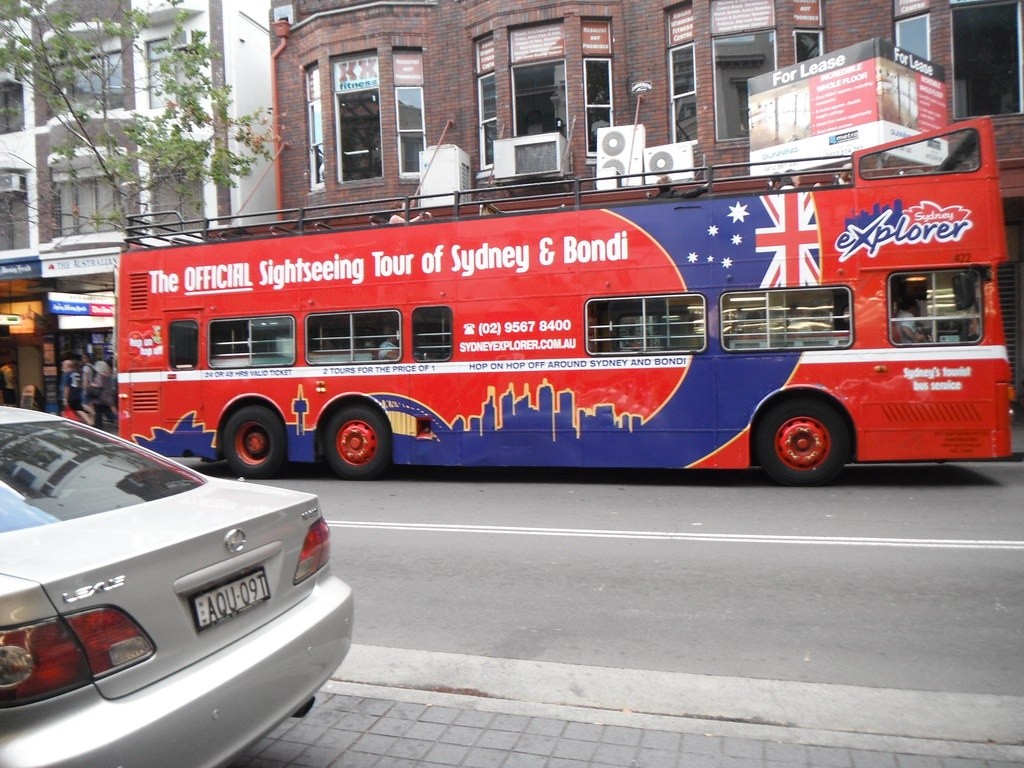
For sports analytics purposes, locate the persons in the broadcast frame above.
[732,311,765,340]
[895,297,928,344]
[378,323,399,360]
[0,360,17,407]
[58,352,119,431]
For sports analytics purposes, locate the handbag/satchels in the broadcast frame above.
[63,405,80,422]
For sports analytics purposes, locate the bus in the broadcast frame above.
[117,115,1011,484]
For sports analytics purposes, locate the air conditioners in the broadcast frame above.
[493,132,569,182]
[644,139,699,183]
[418,144,472,205]
[168,29,193,50]
[0,173,20,192]
[597,124,645,185]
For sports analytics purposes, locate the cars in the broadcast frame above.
[0,407,354,768]
